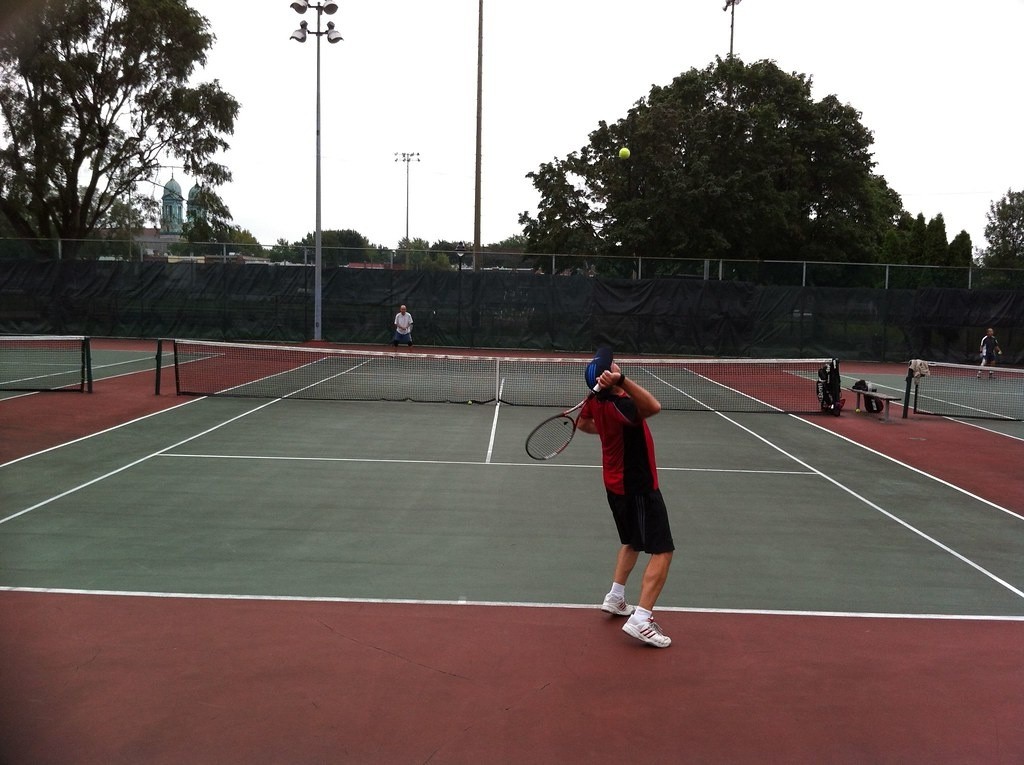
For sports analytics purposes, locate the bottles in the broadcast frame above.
[868,381,872,391]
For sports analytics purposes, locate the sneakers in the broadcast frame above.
[601,593,635,616]
[621,614,671,648]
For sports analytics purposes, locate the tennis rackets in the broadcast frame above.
[525,378,604,460]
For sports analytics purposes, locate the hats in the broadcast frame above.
[585,347,613,390]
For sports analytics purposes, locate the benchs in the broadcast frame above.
[848,387,901,424]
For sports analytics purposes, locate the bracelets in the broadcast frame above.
[615,374,625,387]
[980,351,982,353]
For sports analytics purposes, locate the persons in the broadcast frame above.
[574,345,674,647]
[977,329,1002,378]
[390,305,413,359]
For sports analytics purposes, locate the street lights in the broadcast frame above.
[454,242,467,338]
[395,152,420,270]
[288,0,343,340]
[723,0,742,59]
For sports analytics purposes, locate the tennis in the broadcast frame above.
[998,350,1002,355]
[619,148,630,160]
[856,409,860,413]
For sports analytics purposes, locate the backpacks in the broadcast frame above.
[816,358,845,417]
